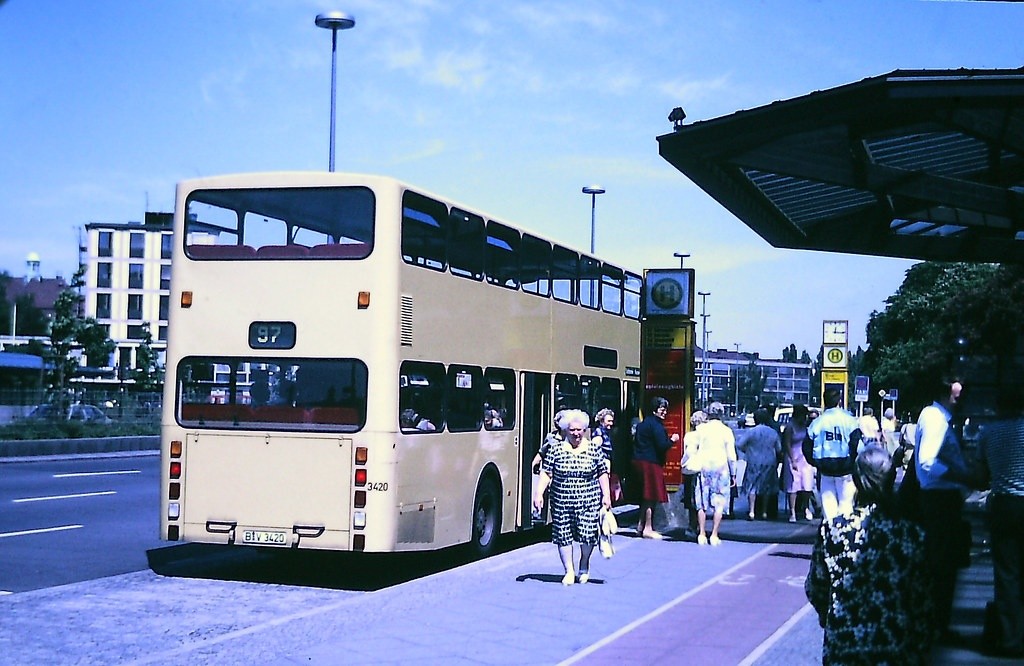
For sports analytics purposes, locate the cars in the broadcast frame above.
[27,405,115,424]
[740,407,767,427]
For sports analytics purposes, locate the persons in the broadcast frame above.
[804,448,934,666]
[534,409,611,585]
[634,398,679,539]
[248,381,507,430]
[592,408,615,480]
[533,411,567,474]
[682,401,917,547]
[914,374,974,646]
[975,389,1024,656]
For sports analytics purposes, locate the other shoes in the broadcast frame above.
[804,507,813,521]
[709,534,724,546]
[683,526,700,540]
[636,527,663,541]
[695,534,708,546]
[788,516,797,524]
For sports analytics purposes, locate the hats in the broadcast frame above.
[701,401,726,420]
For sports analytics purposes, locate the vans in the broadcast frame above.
[774,404,820,432]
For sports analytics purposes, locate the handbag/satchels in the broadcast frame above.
[731,458,747,489]
[597,503,620,560]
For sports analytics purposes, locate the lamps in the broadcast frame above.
[667,107,687,126]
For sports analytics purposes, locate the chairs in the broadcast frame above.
[187,245,256,257]
[253,407,310,423]
[309,407,361,425]
[309,243,371,256]
[256,246,310,257]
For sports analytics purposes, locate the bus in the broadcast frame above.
[160,169,641,562]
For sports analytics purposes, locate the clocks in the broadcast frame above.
[823,320,848,343]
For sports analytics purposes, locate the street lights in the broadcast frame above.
[733,342,742,416]
[675,252,690,267]
[698,289,712,410]
[313,11,358,167]
[581,185,605,309]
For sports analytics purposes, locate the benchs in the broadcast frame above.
[182,403,256,421]
